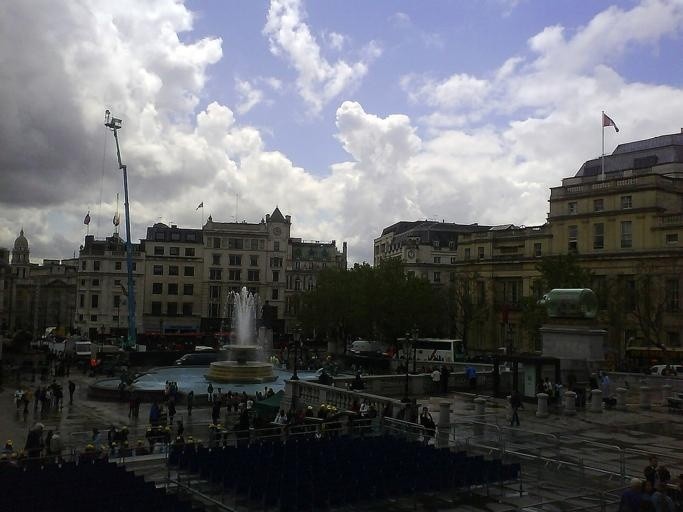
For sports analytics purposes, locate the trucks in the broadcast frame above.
[346,341,376,352]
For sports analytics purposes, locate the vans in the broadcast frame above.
[647,364,683,378]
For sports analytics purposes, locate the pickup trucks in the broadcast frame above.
[496,347,540,355]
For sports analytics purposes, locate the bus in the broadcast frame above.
[63,339,94,362]
[160,334,218,352]
[214,332,293,349]
[396,337,465,362]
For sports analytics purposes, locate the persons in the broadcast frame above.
[0,327,681,510]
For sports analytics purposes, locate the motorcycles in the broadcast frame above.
[11,392,24,408]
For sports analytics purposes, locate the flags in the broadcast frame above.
[603,113,620,132]
[193,199,206,213]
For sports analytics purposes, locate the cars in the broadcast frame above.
[467,354,485,363]
[380,350,394,358]
[488,356,496,363]
[173,353,215,366]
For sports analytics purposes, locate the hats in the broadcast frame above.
[111,427,201,448]
[86,444,95,450]
[307,405,337,411]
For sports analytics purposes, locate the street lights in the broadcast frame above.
[158,311,166,338]
[99,324,104,353]
[290,335,310,380]
[411,323,419,371]
[400,332,411,402]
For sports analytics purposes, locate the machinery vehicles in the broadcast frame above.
[83,105,146,352]
